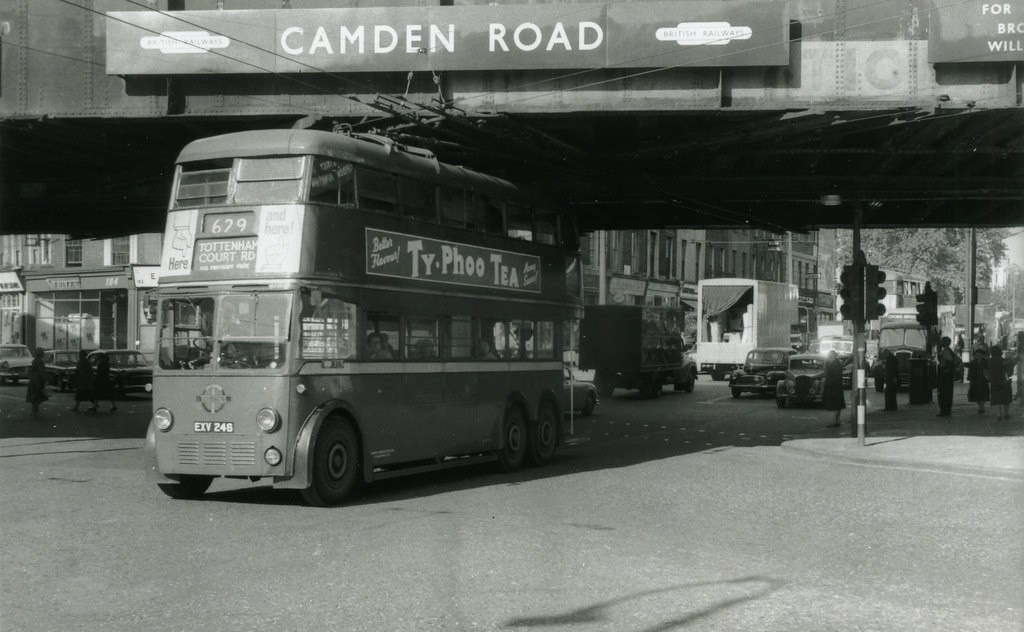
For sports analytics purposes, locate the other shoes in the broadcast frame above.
[997,413,1011,419]
[978,409,986,414]
[937,412,951,417]
[826,423,840,428]
[32,411,41,416]
[111,406,118,411]
[88,407,96,413]
[71,408,79,412]
[882,407,897,411]
[95,404,99,408]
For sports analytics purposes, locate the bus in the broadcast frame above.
[144,127,584,498]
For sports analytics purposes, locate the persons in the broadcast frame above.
[936,337,955,417]
[26,348,48,417]
[882,349,901,411]
[822,350,846,429]
[482,340,499,360]
[967,329,1024,420]
[957,335,964,358]
[69,350,95,412]
[88,352,117,412]
[366,333,439,362]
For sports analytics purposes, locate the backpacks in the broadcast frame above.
[949,354,963,379]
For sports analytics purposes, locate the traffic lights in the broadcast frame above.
[915,293,930,326]
[840,265,859,320]
[867,265,887,321]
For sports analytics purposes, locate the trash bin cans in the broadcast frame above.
[908,358,933,404]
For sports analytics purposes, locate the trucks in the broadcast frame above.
[947,305,997,362]
[871,308,956,390]
[994,311,1011,352]
[697,280,802,381]
[791,305,817,353]
[579,304,699,397]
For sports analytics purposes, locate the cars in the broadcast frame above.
[44,350,85,392]
[865,338,883,374]
[562,366,600,415]
[776,354,828,409]
[817,336,871,387]
[728,345,801,395]
[0,344,38,385]
[84,349,153,397]
[681,336,696,362]
